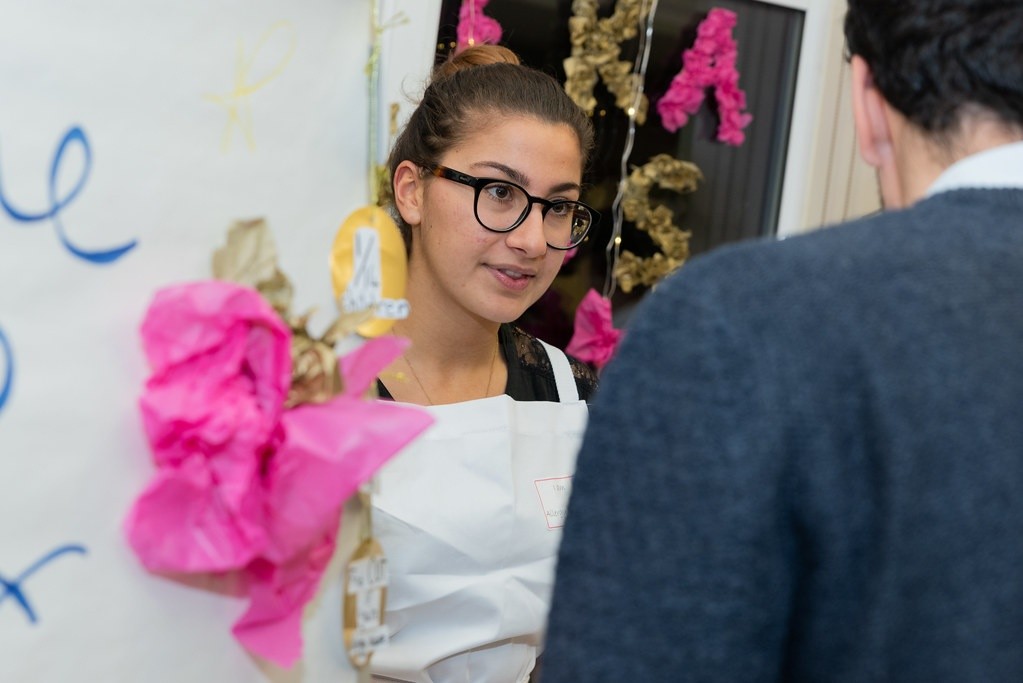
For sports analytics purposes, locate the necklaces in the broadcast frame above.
[390,323,499,405]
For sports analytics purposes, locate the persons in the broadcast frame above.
[530,0,1023,680]
[300,39,601,683]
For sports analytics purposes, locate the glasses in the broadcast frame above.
[432,162,603,252]
